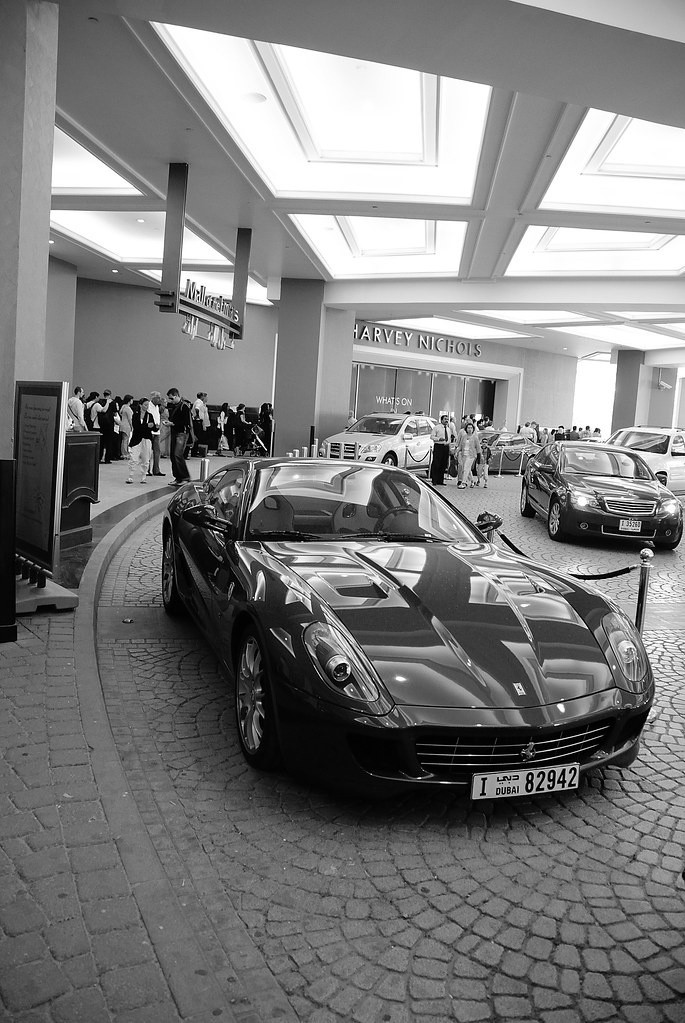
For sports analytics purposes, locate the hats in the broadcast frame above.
[103,389,111,394]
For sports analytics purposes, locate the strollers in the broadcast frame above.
[247,423,269,458]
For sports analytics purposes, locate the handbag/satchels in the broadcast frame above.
[217,435,229,450]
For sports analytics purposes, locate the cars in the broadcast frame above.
[602,427,685,494]
[318,411,451,472]
[519,439,684,552]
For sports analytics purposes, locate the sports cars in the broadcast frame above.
[450,431,543,472]
[159,455,655,802]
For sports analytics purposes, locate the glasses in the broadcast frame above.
[169,396,176,400]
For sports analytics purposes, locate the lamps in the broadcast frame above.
[658,369,672,391]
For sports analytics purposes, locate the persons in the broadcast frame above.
[349,410,357,425]
[125,397,159,484]
[66,386,274,464]
[519,421,601,447]
[162,388,191,485]
[449,414,507,438]
[430,414,451,486]
[146,391,166,476]
[476,438,493,488]
[454,419,484,489]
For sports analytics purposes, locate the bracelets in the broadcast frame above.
[480,456,483,457]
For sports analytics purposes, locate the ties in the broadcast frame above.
[444,426,448,442]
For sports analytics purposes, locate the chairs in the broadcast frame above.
[249,487,294,533]
[333,485,394,535]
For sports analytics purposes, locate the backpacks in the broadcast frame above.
[84,402,98,431]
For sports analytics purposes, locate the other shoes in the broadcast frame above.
[126,478,133,484]
[104,461,111,464]
[168,479,180,485]
[476,481,480,486]
[458,484,466,489]
[145,471,152,476]
[154,472,166,476]
[470,484,474,488]
[176,480,188,485]
[437,483,447,487]
[484,483,488,488]
[140,481,148,484]
